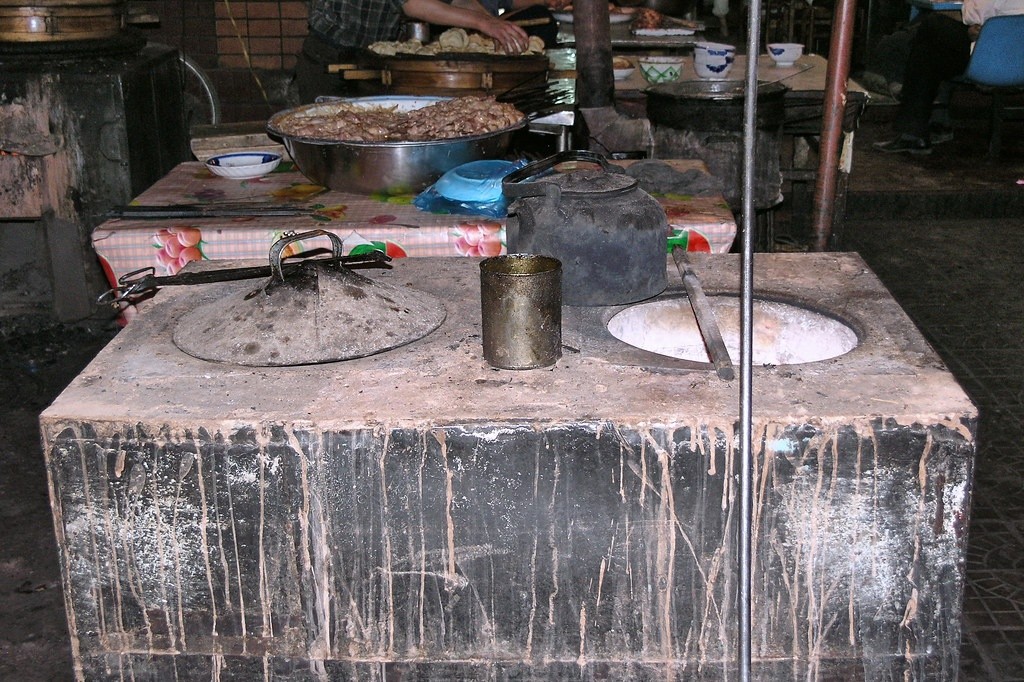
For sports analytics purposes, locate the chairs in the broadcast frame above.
[949,16,1024,161]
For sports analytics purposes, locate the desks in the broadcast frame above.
[91,159,738,324]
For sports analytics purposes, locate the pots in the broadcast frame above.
[644,79,786,130]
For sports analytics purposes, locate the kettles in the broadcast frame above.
[503,150,668,307]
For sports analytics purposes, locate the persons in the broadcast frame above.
[295,1,530,106]
[873,0,1024,156]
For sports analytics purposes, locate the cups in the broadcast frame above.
[480,253,562,370]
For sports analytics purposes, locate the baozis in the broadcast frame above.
[368,28,545,56]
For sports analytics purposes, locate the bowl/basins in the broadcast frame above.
[639,56,684,84]
[766,43,805,65]
[266,95,527,195]
[613,68,636,80]
[693,41,735,79]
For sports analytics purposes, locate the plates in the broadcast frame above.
[205,152,283,179]
[436,160,517,201]
